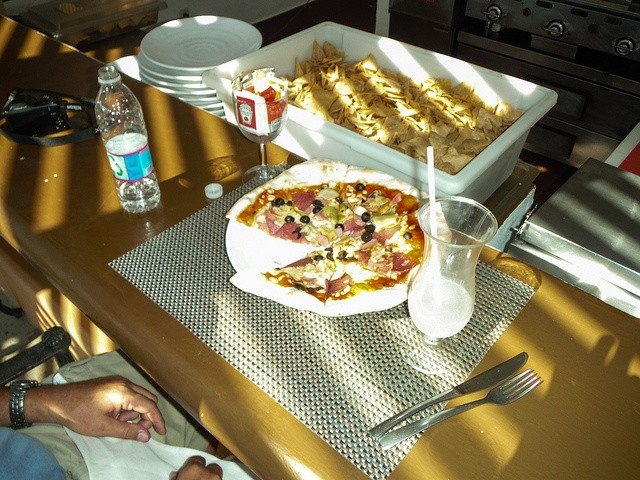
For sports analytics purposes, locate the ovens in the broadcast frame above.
[457,1,639,170]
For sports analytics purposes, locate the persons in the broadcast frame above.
[4,375,223,479]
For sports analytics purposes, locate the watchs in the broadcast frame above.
[9,380,41,430]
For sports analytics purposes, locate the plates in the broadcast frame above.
[206,109,224,116]
[107,55,140,81]
[137,52,203,82]
[219,116,226,120]
[180,96,221,103]
[138,68,212,89]
[140,72,216,95]
[140,15,264,71]
[214,220,321,275]
[195,102,225,110]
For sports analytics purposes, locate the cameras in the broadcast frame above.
[2,96,72,140]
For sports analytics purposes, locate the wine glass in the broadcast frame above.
[232,79,288,191]
[400,195,499,373]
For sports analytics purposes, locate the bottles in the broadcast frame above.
[95,64,161,214]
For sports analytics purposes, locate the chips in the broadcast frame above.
[287,39,522,172]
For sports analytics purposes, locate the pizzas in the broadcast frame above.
[225,159,453,318]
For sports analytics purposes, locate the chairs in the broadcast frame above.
[0,326,75,390]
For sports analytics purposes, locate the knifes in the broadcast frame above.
[369,351,528,460]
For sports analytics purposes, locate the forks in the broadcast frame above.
[378,369,541,451]
